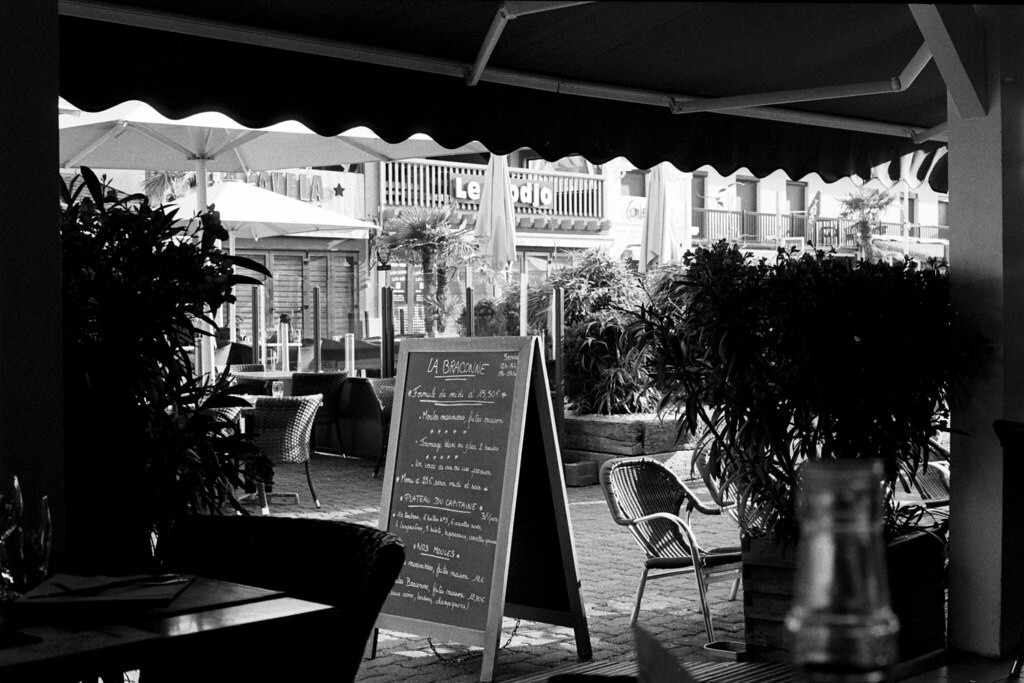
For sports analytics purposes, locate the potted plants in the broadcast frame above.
[603,231,967,646]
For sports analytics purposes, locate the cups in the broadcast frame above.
[272,381,284,397]
[6,494,52,580]
[293,330,301,342]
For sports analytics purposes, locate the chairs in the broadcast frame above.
[192,362,396,512]
[911,463,950,517]
[151,510,405,682]
[599,456,751,644]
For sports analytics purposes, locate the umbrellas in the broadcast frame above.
[474,152,518,298]
[56,90,531,394]
[638,161,681,271]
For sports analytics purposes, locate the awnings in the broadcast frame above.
[52,0,1004,683]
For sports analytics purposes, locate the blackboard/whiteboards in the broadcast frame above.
[374,330,587,648]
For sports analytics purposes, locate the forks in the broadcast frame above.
[50,579,188,594]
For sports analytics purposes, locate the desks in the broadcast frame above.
[230,371,297,399]
[0,561,334,681]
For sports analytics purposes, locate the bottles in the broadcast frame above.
[785,457,900,682]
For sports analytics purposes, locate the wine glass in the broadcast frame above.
[139,534,187,585]
[0,474,25,601]
